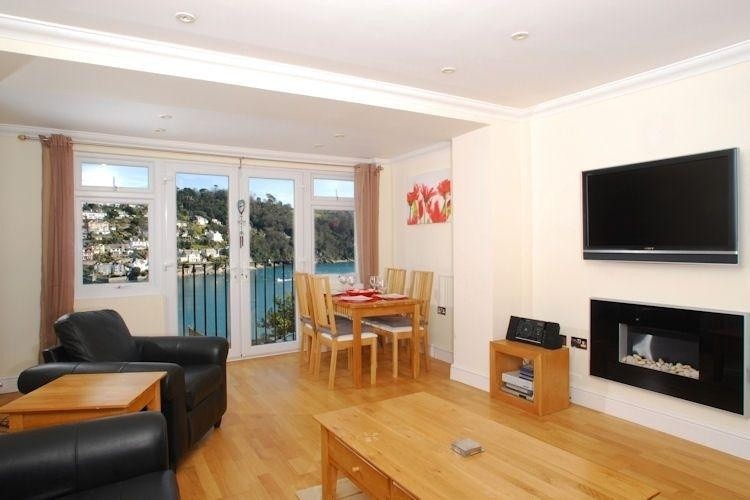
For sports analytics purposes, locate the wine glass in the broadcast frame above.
[369,275,380,296]
[347,274,356,291]
[338,274,349,295]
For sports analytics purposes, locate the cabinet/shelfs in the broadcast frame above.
[489,339,572,416]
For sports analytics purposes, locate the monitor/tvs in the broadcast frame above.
[580,148,740,267]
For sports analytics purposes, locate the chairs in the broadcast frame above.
[293,266,435,390]
[0,409,181,500]
[14,308,229,472]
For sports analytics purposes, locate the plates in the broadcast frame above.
[323,289,345,296]
[338,295,373,303]
[374,293,408,300]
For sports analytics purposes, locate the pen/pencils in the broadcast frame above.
[647,493,660,500]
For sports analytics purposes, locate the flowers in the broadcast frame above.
[403,178,451,225]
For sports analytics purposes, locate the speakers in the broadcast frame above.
[506,316,520,342]
[542,322,560,349]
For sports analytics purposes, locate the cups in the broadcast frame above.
[377,278,387,295]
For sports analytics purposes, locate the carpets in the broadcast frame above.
[295,477,373,500]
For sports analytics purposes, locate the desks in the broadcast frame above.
[0,369,168,430]
[313,392,663,500]
